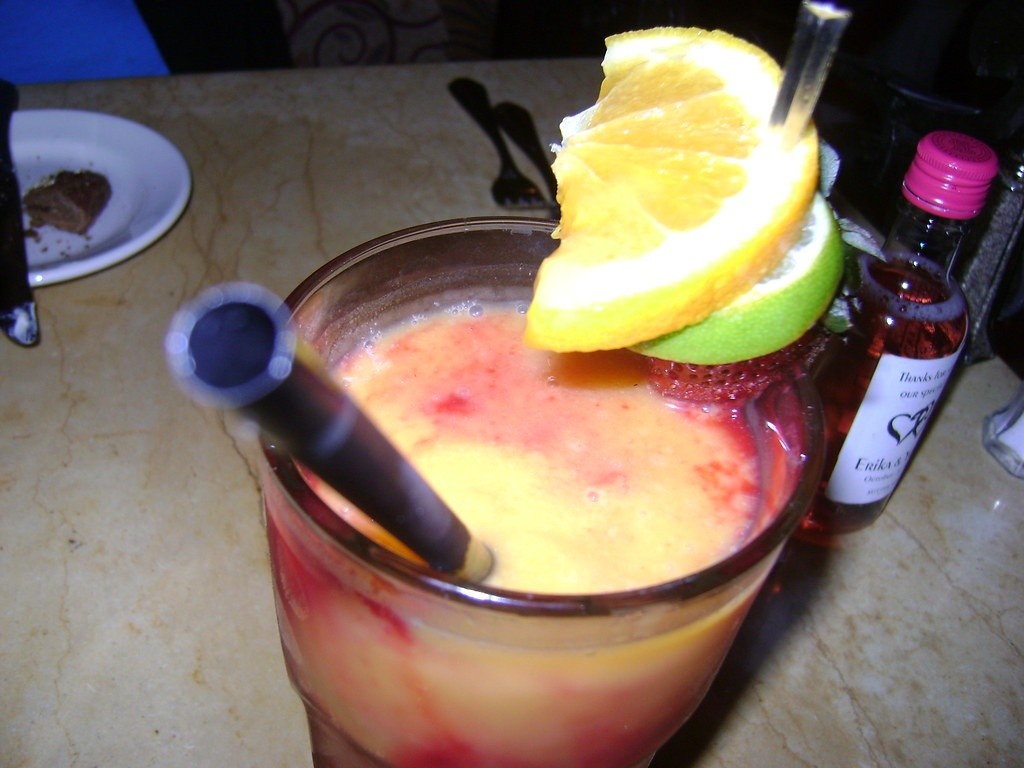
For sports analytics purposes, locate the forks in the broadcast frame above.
[446,76,548,210]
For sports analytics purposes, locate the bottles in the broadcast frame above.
[951,125,1024,366]
[792,130,999,536]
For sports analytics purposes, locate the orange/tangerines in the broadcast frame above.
[522,26,821,351]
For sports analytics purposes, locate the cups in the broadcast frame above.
[260,216,824,768]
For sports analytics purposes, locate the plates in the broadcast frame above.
[8,107,193,288]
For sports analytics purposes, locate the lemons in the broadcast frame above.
[625,190,844,364]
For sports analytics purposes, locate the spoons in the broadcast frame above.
[0,77,40,348]
